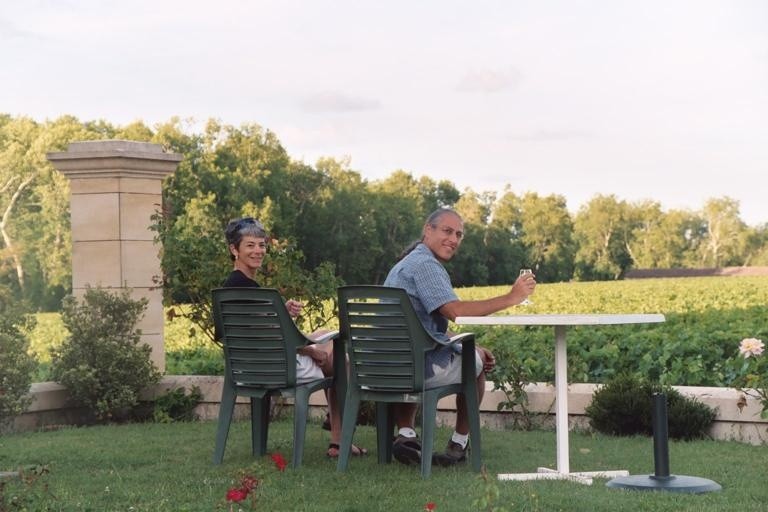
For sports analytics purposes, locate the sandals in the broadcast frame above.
[328,443,369,458]
[321,412,331,431]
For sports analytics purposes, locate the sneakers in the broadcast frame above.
[392,434,455,468]
[445,438,471,461]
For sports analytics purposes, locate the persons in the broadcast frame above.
[211,215,369,459]
[363,208,539,468]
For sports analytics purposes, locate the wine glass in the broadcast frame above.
[519,269,534,306]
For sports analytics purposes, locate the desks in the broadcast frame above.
[454,312,666,488]
